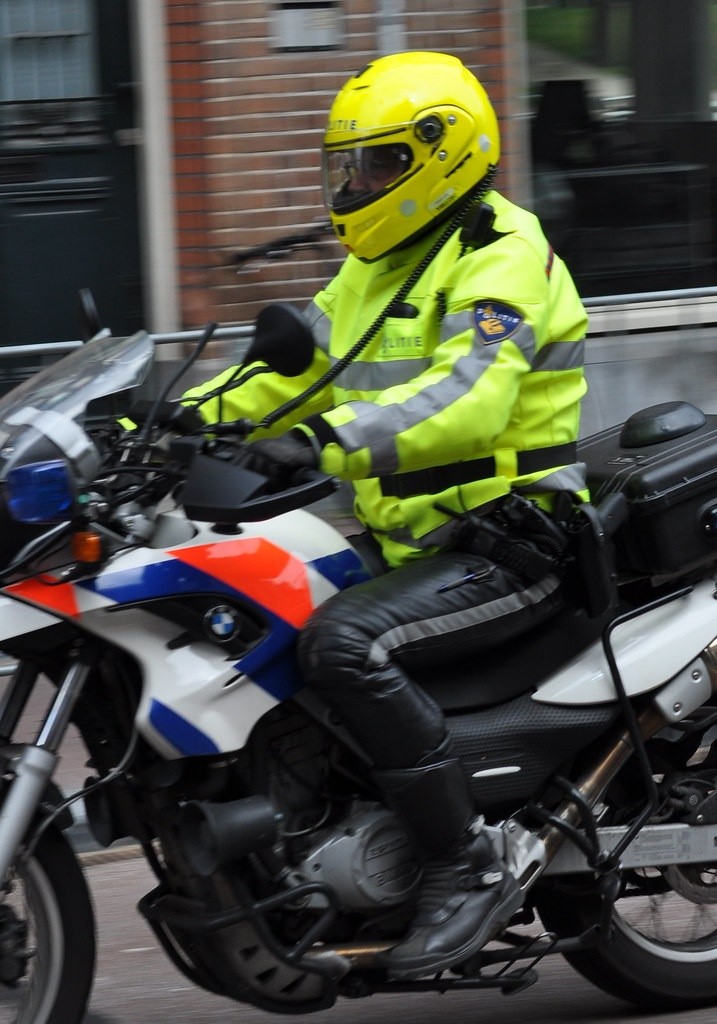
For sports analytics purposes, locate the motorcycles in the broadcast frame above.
[0,289,717,1024]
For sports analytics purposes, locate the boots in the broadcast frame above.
[371,728,526,981]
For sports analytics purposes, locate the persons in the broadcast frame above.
[115,50,610,978]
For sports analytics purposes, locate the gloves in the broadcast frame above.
[231,430,316,491]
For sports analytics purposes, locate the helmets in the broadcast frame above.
[321,51,501,264]
